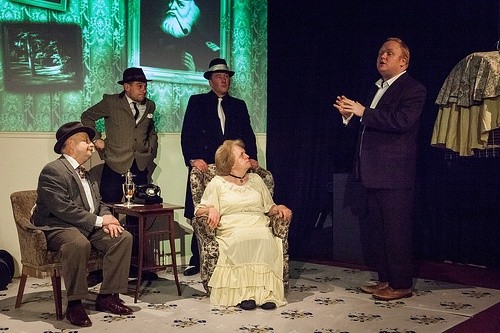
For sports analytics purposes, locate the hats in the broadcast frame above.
[54,121,96,154]
[204,58,235,80]
[117,68,152,84]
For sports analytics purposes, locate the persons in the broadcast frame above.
[192,140,293,312]
[181,60,260,275]
[80,68,160,286]
[332,37,427,300]
[34,122,134,327]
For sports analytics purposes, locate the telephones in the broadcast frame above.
[132,183,164,204]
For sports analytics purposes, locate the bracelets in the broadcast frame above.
[92,138,98,144]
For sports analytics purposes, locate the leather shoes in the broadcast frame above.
[360,282,390,294]
[66,303,92,327]
[95,293,133,314]
[372,286,412,300]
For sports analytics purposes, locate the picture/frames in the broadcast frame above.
[126,0,235,84]
[10,0,69,12]
[2,23,84,92]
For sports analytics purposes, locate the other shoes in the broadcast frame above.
[241,299,256,310]
[260,301,276,309]
[183,265,198,276]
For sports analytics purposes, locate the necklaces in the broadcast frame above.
[228,172,247,183]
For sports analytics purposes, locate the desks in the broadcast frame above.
[105,202,185,304]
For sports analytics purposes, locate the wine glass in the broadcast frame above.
[122,183,136,206]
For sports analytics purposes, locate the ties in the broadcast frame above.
[217,97,225,135]
[78,166,86,178]
[131,102,139,121]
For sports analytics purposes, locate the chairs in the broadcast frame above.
[10,188,112,320]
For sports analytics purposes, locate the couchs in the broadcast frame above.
[189,162,290,298]
